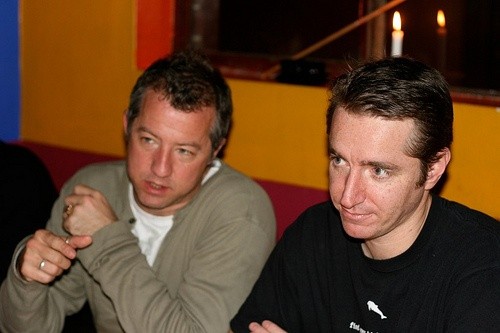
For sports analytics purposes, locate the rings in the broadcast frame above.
[39,258,47,270]
[65,235,70,244]
[63,201,75,215]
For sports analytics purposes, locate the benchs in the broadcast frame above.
[8,140,330,245]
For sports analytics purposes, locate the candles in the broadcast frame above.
[389,10,406,58]
[433,9,447,71]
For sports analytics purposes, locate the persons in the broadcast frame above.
[0,48,278,333]
[228,54,496,333]
[0,141,98,333]
